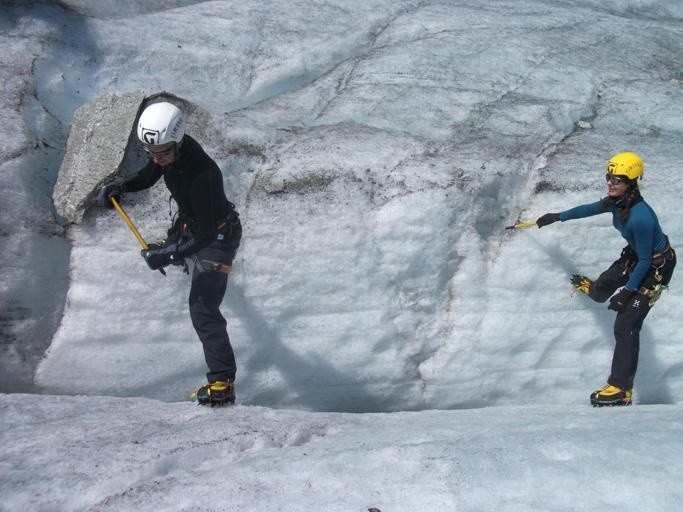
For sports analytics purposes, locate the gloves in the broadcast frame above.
[536,213,560,229]
[141,243,183,270]
[96,177,127,209]
[607,288,634,312]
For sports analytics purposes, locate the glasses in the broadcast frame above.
[143,146,170,159]
[605,173,621,185]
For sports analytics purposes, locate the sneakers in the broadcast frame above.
[196,380,234,406]
[589,384,632,407]
[570,275,592,294]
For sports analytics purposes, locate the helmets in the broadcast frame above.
[137,101,186,147]
[605,151,644,180]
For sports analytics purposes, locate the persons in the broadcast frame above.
[535,151,677,407]
[96,102,242,408]
